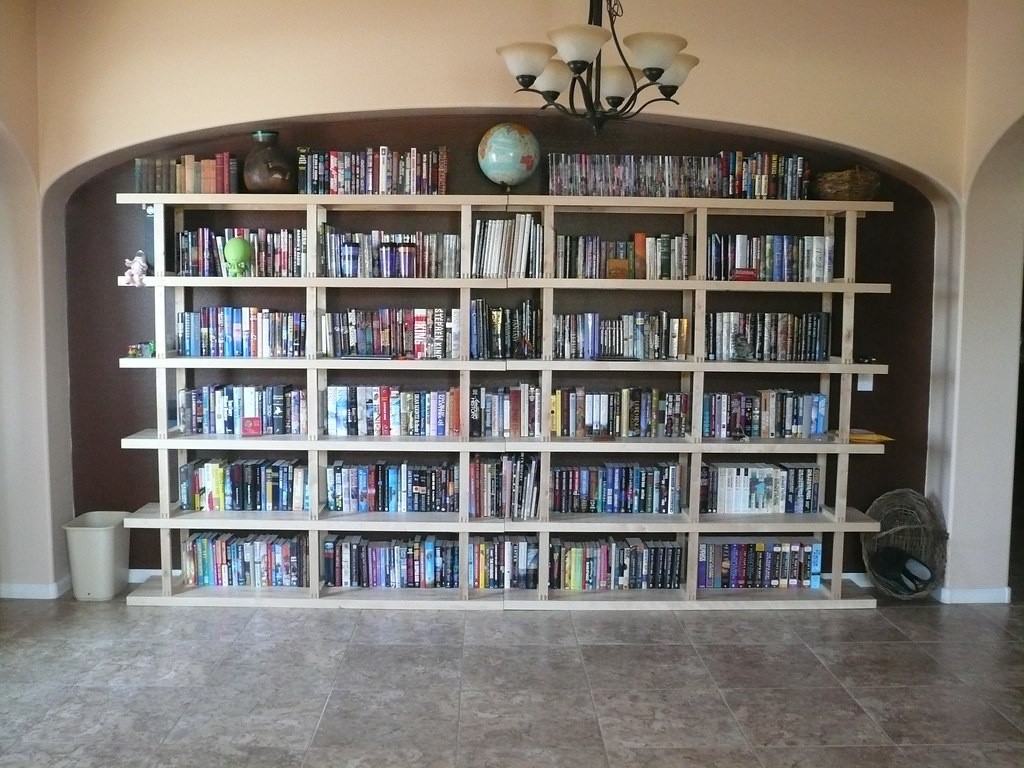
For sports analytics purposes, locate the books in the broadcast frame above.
[135,148,833,588]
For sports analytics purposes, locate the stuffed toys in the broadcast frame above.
[124,250,148,288]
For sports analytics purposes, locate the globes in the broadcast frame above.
[476,122,541,195]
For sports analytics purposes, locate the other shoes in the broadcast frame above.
[881,547,933,586]
[868,552,918,595]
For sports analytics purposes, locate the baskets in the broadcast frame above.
[860,488,949,601]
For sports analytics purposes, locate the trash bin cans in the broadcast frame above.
[62,510,129,604]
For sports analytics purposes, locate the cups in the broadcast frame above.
[398,243,416,278]
[378,243,398,278]
[343,243,359,278]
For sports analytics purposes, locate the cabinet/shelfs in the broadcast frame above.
[116,193,895,611]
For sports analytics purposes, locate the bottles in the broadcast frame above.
[420,333,437,360]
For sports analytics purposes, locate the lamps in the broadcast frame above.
[494,0,700,137]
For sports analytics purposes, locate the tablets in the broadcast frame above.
[341,354,394,360]
